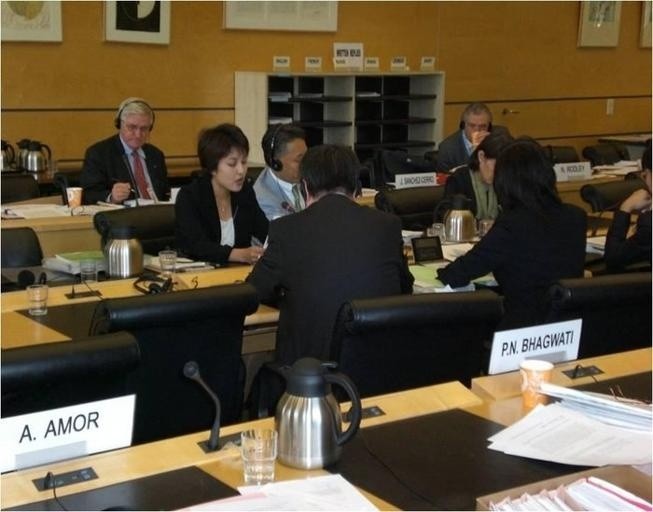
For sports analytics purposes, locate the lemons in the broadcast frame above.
[1,138,52,173]
[100,223,144,278]
[434,194,475,242]
[274,357,362,469]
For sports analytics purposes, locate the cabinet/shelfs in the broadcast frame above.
[234,69,445,164]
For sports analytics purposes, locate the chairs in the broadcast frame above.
[2,144,652,447]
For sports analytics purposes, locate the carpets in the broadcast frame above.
[292,185,301,213]
[132,150,151,199]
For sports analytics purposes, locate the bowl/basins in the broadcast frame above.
[214,197,232,224]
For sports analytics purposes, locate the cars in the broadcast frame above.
[471,132,482,151]
[66,188,83,208]
[479,220,494,239]
[518,360,554,410]
[80,258,98,286]
[26,284,49,316]
[240,427,278,487]
[426,222,446,245]
[159,250,177,281]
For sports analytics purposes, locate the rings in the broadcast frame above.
[112,178,135,193]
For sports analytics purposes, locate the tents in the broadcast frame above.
[134,274,172,294]
[269,123,294,171]
[460,102,493,132]
[114,101,155,131]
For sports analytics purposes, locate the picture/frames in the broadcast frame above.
[638,1,652,50]
[472,346,652,407]
[577,2,624,48]
[598,134,652,159]
[0,379,600,510]
[102,1,172,46]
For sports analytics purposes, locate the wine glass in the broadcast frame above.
[258,255,261,259]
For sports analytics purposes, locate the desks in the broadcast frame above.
[0,379,600,510]
[472,346,652,407]
[598,134,652,159]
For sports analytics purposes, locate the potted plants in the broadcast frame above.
[638,1,652,50]
[102,1,172,46]
[577,2,624,48]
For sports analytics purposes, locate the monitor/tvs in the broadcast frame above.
[47,250,105,275]
[151,257,215,272]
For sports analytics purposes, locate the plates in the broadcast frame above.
[234,69,445,164]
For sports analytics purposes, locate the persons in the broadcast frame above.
[246,145,414,366]
[251,124,307,222]
[175,124,270,265]
[605,143,652,262]
[445,133,514,235]
[77,96,169,204]
[435,136,588,332]
[437,103,512,174]
